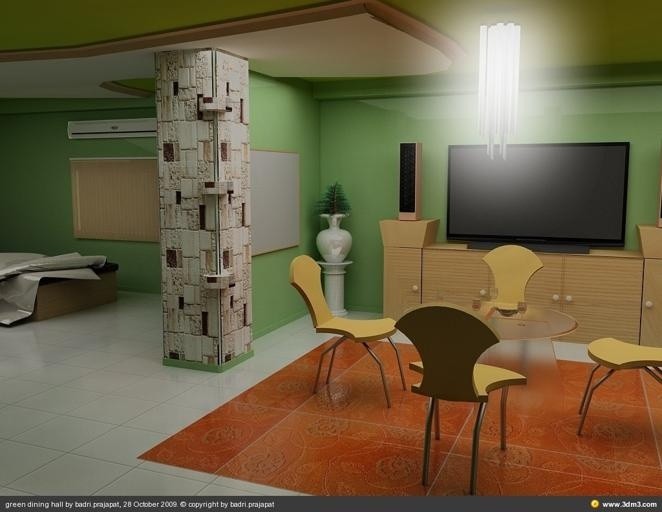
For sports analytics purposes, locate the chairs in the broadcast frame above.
[482,245,543,302]
[394,302,527,496]
[577,336,661,436]
[289,255,406,409]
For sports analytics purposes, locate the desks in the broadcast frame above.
[409,300,578,416]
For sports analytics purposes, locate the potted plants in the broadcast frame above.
[313,181,353,263]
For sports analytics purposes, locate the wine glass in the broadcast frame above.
[518,302,527,326]
[472,300,481,317]
[489,287,498,302]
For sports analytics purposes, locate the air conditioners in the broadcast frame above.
[67,117,159,140]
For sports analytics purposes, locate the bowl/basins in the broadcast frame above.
[497,309,519,317]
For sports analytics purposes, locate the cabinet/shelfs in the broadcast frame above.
[423,249,643,369]
[383,245,422,321]
[640,257,662,350]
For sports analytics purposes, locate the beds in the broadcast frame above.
[1,252,118,324]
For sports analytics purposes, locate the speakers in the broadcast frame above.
[397,141,422,221]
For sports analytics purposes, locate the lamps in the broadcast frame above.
[479,22,521,161]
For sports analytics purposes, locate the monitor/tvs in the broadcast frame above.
[447,142,630,254]
